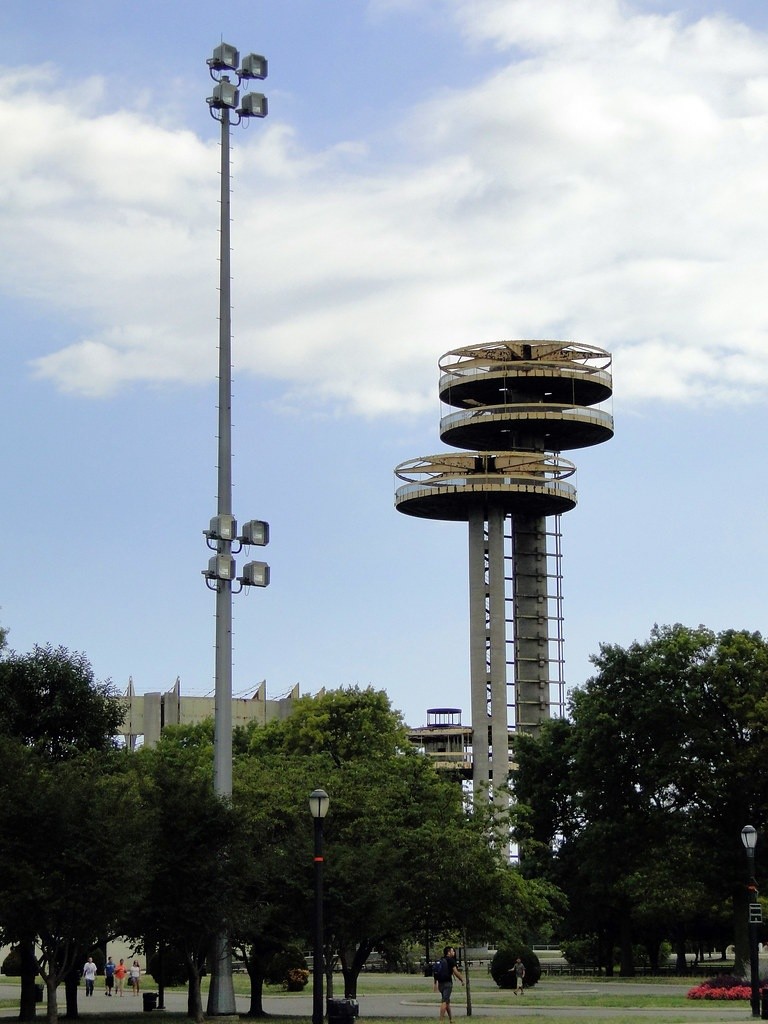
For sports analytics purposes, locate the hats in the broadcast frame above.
[516,959,521,962]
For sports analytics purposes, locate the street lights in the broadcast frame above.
[740,825,762,1016]
[307,787,330,1024]
[198,33,273,1016]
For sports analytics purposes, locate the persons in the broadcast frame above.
[433,946,465,1024]
[508,959,525,995]
[84,957,141,997]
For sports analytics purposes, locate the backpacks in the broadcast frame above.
[432,956,453,980]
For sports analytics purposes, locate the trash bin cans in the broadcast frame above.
[142,992,157,1011]
[35,983,44,1003]
[761,988,768,1020]
[325,997,359,1024]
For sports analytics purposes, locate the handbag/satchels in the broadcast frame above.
[127,978,133,986]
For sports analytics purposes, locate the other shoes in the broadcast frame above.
[514,992,517,995]
[105,993,107,995]
[109,994,111,996]
[521,993,524,995]
